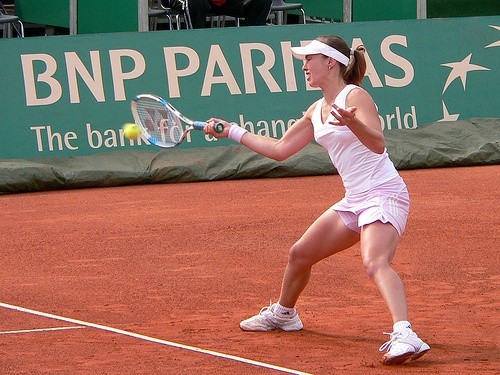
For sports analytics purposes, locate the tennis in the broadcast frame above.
[124,123,141,140]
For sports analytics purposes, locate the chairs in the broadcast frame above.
[0,4,25,38]
[148,9,172,30]
[270,1,306,24]
[162,0,186,30]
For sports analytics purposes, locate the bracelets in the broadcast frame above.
[228,124,247,144]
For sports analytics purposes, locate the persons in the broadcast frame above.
[204,34,432,366]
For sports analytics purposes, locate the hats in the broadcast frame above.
[289,39,349,66]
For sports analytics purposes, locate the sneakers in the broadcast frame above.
[379,328,431,365]
[239,300,304,332]
